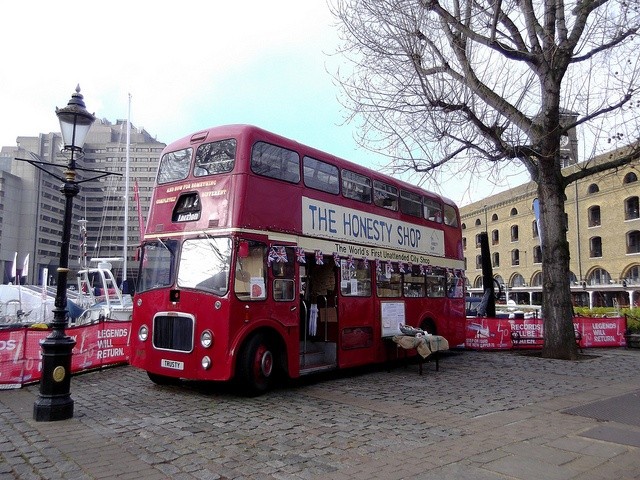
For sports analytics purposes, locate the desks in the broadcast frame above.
[392,335,449,375]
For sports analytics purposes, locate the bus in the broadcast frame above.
[128,124,466,397]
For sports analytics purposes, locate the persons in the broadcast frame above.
[507,296,517,318]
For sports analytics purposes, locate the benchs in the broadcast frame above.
[511,330,583,353]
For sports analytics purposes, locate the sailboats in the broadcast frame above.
[65,93,148,327]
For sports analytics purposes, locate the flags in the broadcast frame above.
[295,247,306,264]
[426,265,432,273]
[347,255,354,269]
[397,261,406,273]
[275,247,288,263]
[315,250,324,265]
[418,265,425,276]
[361,257,368,270]
[332,252,341,268]
[386,260,394,273]
[407,262,413,272]
[267,247,280,267]
[375,259,382,271]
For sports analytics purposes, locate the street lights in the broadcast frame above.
[15,83,123,421]
